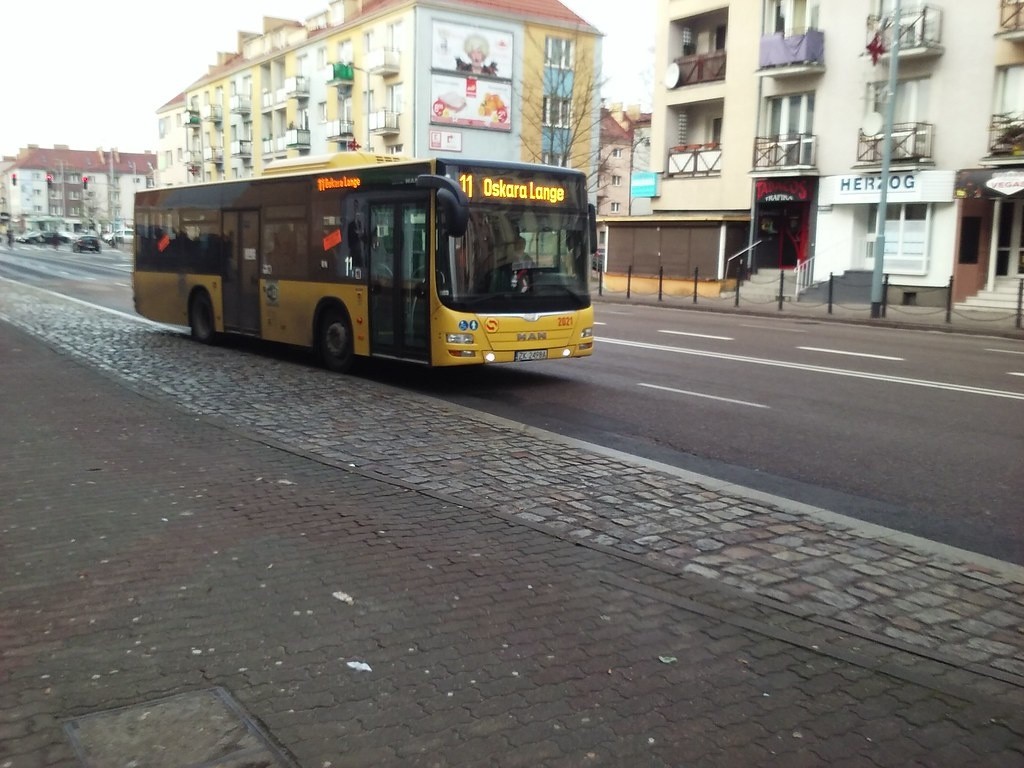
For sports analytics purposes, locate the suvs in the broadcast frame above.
[102,227,134,245]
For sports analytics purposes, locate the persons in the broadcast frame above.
[513,237,532,261]
[456,34,499,76]
[7,225,14,244]
[457,236,489,274]
[40,228,46,244]
[53,228,60,247]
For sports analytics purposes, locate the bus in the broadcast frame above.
[131,153,600,384]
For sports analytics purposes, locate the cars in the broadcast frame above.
[72,235,102,254]
[14,229,71,247]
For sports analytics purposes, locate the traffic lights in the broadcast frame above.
[82,176,88,189]
[12,174,17,186]
[47,174,53,189]
